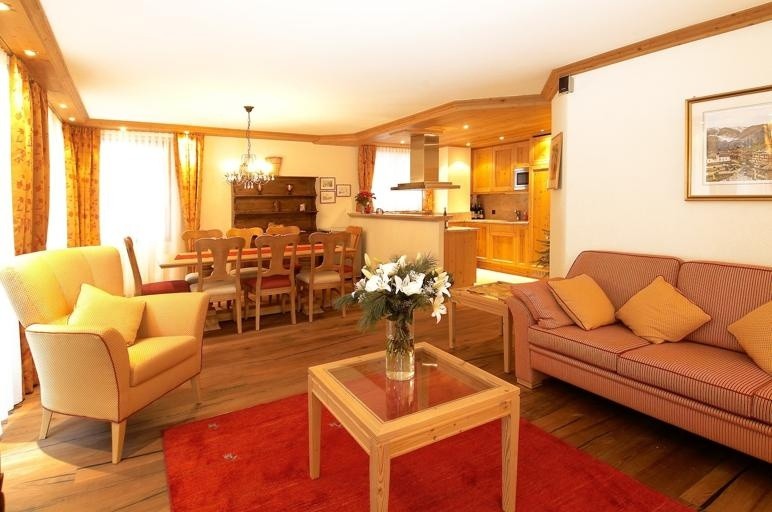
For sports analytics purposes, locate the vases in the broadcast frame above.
[383,314,417,382]
[362,203,370,214]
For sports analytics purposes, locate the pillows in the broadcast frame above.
[69,282,146,347]
[510,276,575,331]
[545,272,617,332]
[613,276,711,343]
[726,301,771,374]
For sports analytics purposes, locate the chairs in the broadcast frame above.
[124,225,365,335]
[0,243,209,465]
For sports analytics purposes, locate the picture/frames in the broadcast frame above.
[549,131,565,192]
[318,177,352,203]
[684,85,771,202]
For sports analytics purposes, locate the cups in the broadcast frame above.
[300,203,305,211]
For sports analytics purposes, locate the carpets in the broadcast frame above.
[162,360,704,512]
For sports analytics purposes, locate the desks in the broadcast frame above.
[446,281,520,375]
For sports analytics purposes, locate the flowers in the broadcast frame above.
[328,251,455,356]
[355,188,376,204]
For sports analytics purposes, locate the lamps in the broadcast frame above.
[224,107,275,190]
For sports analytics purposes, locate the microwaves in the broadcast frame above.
[513,167,529,191]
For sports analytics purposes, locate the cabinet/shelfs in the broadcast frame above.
[229,174,319,234]
[473,147,494,193]
[459,222,488,262]
[530,135,550,269]
[492,139,530,193]
[487,222,528,268]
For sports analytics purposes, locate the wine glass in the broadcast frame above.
[257,183,263,195]
[286,184,293,195]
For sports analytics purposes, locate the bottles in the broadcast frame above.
[470,201,483,219]
[443,208,448,229]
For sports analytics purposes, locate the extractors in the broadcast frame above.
[390,134,461,190]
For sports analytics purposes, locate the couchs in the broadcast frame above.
[506,249,772,464]
